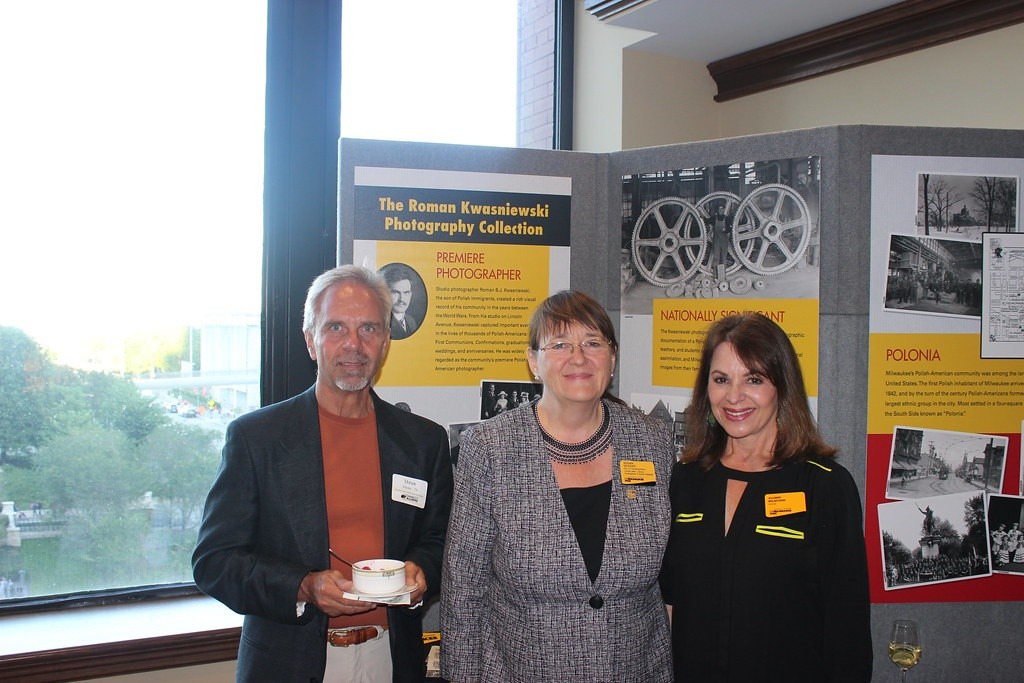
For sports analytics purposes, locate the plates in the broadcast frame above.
[342,582,419,598]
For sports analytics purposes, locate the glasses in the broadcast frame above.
[532,337,615,352]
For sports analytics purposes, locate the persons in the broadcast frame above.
[384,268,417,338]
[451,428,467,473]
[919,506,935,532]
[901,471,976,487]
[991,523,1024,566]
[191,266,455,683]
[13,504,42,519]
[888,555,988,586]
[486,384,540,419]
[887,272,981,308]
[658,313,873,683]
[440,290,675,683]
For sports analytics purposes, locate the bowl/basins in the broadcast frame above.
[352,558,406,594]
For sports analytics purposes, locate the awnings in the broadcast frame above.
[892,461,922,470]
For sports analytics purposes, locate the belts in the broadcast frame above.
[325,626,388,647]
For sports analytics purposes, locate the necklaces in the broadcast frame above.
[531,398,614,464]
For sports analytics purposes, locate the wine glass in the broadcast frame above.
[888,620,921,683]
